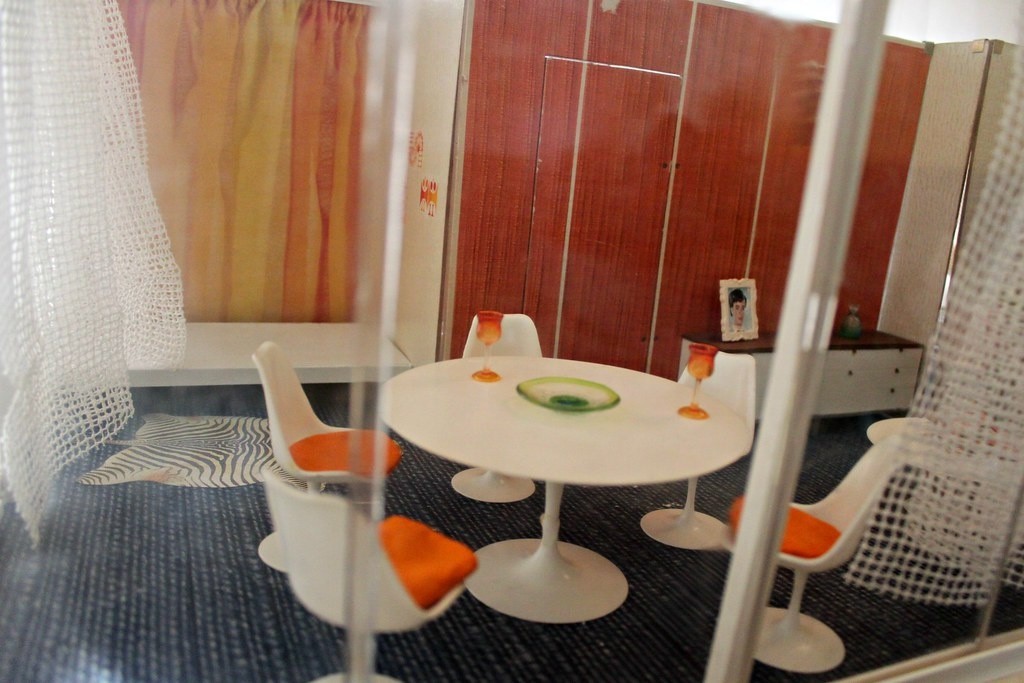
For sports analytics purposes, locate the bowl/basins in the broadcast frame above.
[516,376,621,427]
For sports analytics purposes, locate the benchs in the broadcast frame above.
[37,315,412,391]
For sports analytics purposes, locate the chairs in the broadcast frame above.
[258,466,477,682]
[251,341,401,572]
[640,340,757,549]
[450,311,548,501]
[729,433,908,674]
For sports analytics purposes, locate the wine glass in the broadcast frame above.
[471,311,504,382]
[677,342,719,421]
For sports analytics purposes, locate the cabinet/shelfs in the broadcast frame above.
[679,329,927,434]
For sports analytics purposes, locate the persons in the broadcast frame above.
[729,289,746,333]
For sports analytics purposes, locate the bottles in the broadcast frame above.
[840,304,861,339]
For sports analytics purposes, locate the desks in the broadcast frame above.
[867,418,1022,562]
[378,355,750,622]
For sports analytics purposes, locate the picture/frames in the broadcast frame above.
[719,278,760,342]
